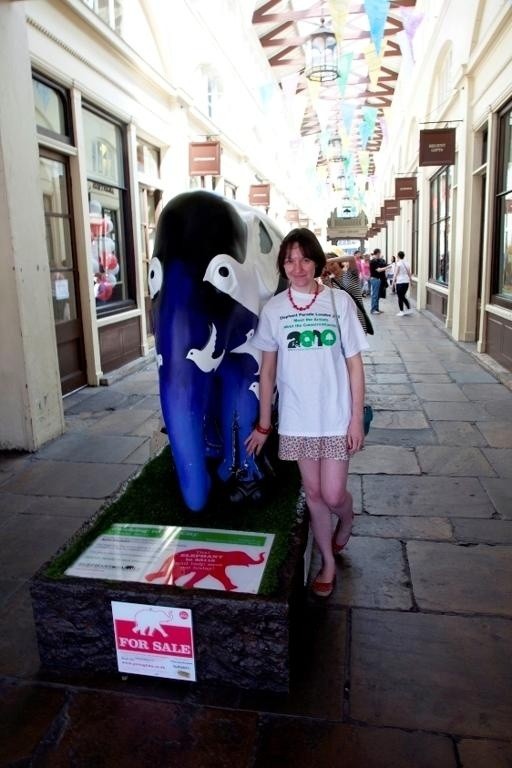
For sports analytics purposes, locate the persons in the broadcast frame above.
[325,249,413,337]
[243,226,369,599]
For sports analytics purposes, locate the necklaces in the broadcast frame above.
[288,279,318,311]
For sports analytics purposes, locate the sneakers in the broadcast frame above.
[312,566,336,596]
[332,510,354,551]
[372,309,413,317]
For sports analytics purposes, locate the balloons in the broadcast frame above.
[89,203,120,302]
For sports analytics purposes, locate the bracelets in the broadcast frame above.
[255,424,272,434]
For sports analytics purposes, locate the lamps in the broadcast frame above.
[328,120,352,219]
[305,0,339,83]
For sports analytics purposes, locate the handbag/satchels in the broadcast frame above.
[363,312,374,334]
[391,283,395,292]
